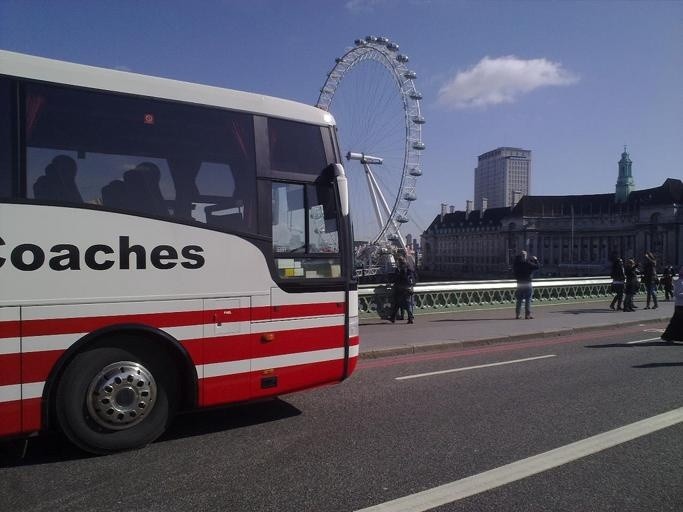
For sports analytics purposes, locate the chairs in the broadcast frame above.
[33,165,172,217]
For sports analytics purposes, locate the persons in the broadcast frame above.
[627,256,638,309]
[661,263,683,341]
[624,258,638,313]
[390,256,417,324]
[609,257,625,311]
[660,266,674,299]
[394,258,413,320]
[55,156,84,205]
[514,250,541,319]
[642,250,659,309]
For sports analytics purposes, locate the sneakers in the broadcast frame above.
[516,314,535,320]
[610,303,657,312]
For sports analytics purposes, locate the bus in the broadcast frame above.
[0,48,361,456]
